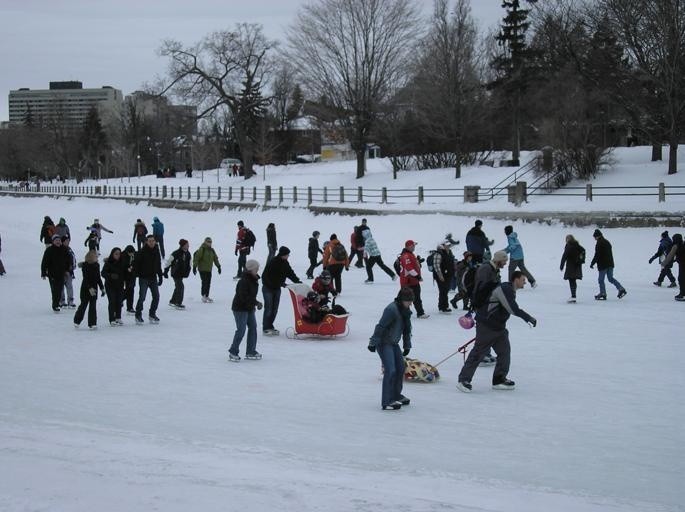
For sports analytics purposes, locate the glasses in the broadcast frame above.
[206,240,213,243]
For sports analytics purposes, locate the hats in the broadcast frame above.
[405,239,418,248]
[397,287,417,303]
[179,238,189,246]
[245,258,260,271]
[51,233,62,242]
[593,228,603,237]
[463,251,473,259]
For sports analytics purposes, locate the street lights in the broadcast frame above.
[26,135,154,181]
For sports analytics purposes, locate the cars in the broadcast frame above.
[218,158,241,168]
[294,153,322,163]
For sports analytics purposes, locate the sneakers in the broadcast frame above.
[380,394,410,410]
[456,378,473,393]
[492,378,516,390]
[478,354,499,368]
[261,326,280,336]
[228,350,241,362]
[246,352,263,359]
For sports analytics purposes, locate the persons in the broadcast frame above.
[227,164,232,176]
[261,246,303,337]
[156,164,193,178]
[233,164,238,176]
[465,220,494,255]
[661,234,685,300]
[133,218,148,251]
[73,249,105,330]
[228,259,263,359]
[399,240,431,320]
[134,234,162,324]
[458,270,538,390]
[432,234,483,315]
[472,250,509,364]
[233,220,250,279]
[228,259,263,362]
[501,225,536,287]
[367,286,413,408]
[164,239,192,310]
[193,237,221,303]
[306,231,350,323]
[0,236,5,275]
[84,218,113,255]
[40,215,77,313]
[648,230,677,288]
[266,223,278,262]
[560,235,587,302]
[151,217,165,259]
[349,218,398,283]
[120,245,135,312]
[588,228,627,300]
[101,247,124,327]
